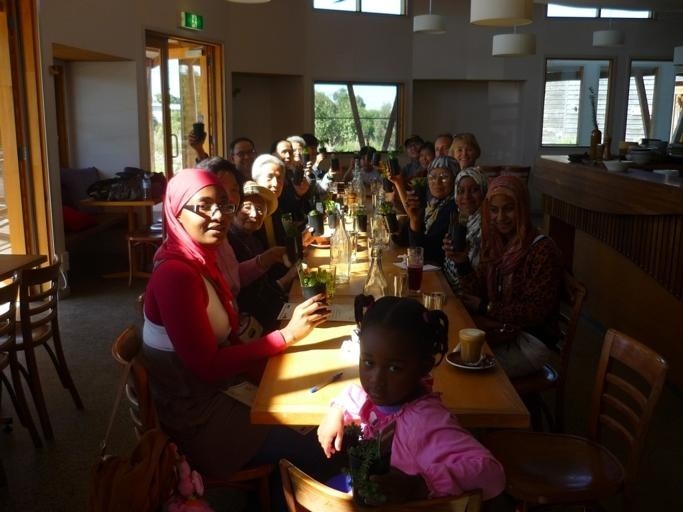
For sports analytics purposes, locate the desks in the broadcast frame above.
[0,253,48,442]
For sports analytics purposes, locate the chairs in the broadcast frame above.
[110,323,276,511]
[278,458,484,512]
[0,270,44,452]
[125,206,163,290]
[0,252,85,444]
[509,272,589,431]
[488,330,672,512]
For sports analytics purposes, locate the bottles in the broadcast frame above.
[370,190,390,244]
[349,159,367,202]
[590,124,608,161]
[364,247,388,298]
[142,173,152,201]
[331,218,351,282]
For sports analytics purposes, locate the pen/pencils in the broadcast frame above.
[310,372,344,394]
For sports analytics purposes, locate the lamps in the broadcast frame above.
[592,19,625,48]
[469,0,537,58]
[412,0,447,36]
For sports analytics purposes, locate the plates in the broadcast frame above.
[445,350,496,371]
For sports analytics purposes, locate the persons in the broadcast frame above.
[141,131,556,511]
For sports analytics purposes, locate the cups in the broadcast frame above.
[448,221,466,253]
[391,247,424,298]
[412,185,427,209]
[423,291,446,309]
[331,157,340,172]
[327,214,337,228]
[286,234,303,261]
[357,215,367,231]
[308,214,324,236]
[302,266,336,317]
[192,123,205,143]
[459,329,485,364]
[386,157,400,175]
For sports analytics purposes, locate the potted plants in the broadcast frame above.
[588,86,602,151]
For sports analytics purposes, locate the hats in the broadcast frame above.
[243,180,280,219]
[404,135,424,145]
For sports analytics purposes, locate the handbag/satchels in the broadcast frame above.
[91,435,180,512]
[87,167,167,201]
[491,331,553,381]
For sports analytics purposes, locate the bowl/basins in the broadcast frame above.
[603,160,631,171]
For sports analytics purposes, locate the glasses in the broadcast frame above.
[427,172,452,184]
[231,148,257,159]
[182,202,237,218]
